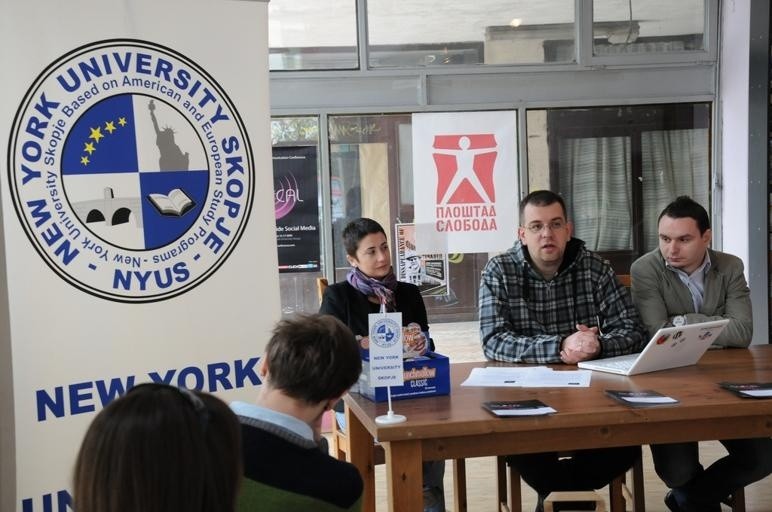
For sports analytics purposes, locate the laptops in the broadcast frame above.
[577,318,730,376]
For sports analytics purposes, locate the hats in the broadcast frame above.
[405,251,420,259]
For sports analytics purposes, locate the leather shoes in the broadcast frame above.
[664,491,680,512]
[723,493,736,512]
[535,495,557,512]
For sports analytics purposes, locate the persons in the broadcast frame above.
[318,218,434,362]
[478,191,649,363]
[229,314,363,512]
[631,196,753,350]
[70,383,244,512]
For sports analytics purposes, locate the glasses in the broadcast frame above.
[521,221,563,233]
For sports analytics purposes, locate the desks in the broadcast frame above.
[337,343,772,512]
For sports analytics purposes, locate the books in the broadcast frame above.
[481,399,559,419]
[605,388,679,407]
[719,381,772,399]
